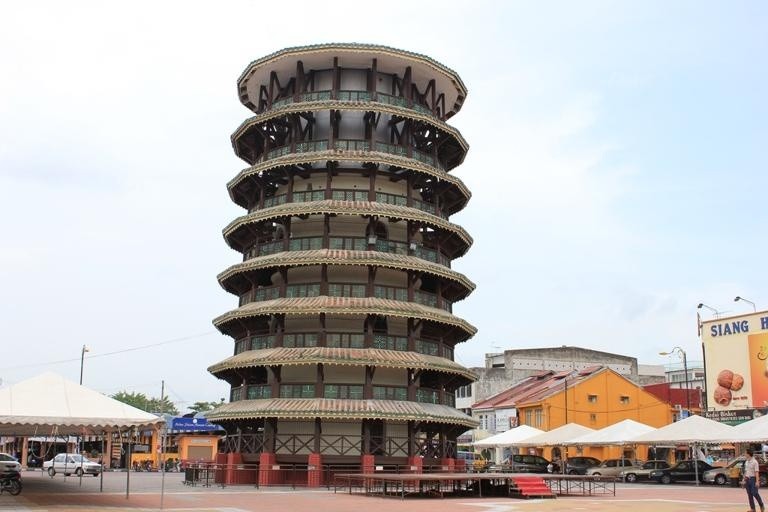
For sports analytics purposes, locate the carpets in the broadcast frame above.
[510,476,554,496]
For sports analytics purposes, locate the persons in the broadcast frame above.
[546,462,553,473]
[743,448,766,512]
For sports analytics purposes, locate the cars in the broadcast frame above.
[457,452,495,472]
[43,453,102,477]
[585,456,768,485]
[0,452,22,475]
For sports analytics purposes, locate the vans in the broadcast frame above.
[565,457,601,475]
[509,455,560,473]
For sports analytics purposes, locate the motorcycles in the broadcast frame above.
[0,464,23,497]
